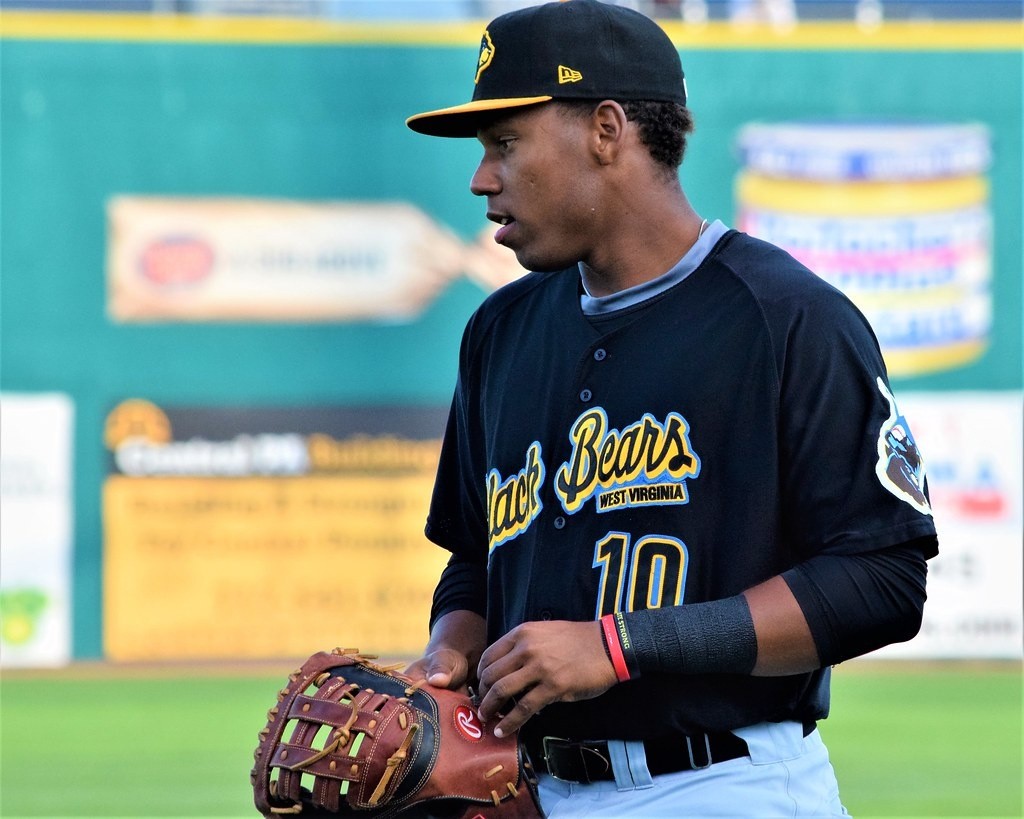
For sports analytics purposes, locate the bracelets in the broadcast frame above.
[601,611,643,682]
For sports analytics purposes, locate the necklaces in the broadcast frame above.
[581,218,707,298]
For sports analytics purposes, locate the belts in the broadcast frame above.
[517,719,817,785]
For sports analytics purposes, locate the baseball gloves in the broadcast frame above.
[248,646,552,819]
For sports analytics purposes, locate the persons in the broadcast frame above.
[401,0,939,816]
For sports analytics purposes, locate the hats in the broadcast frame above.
[405,0,686,138]
